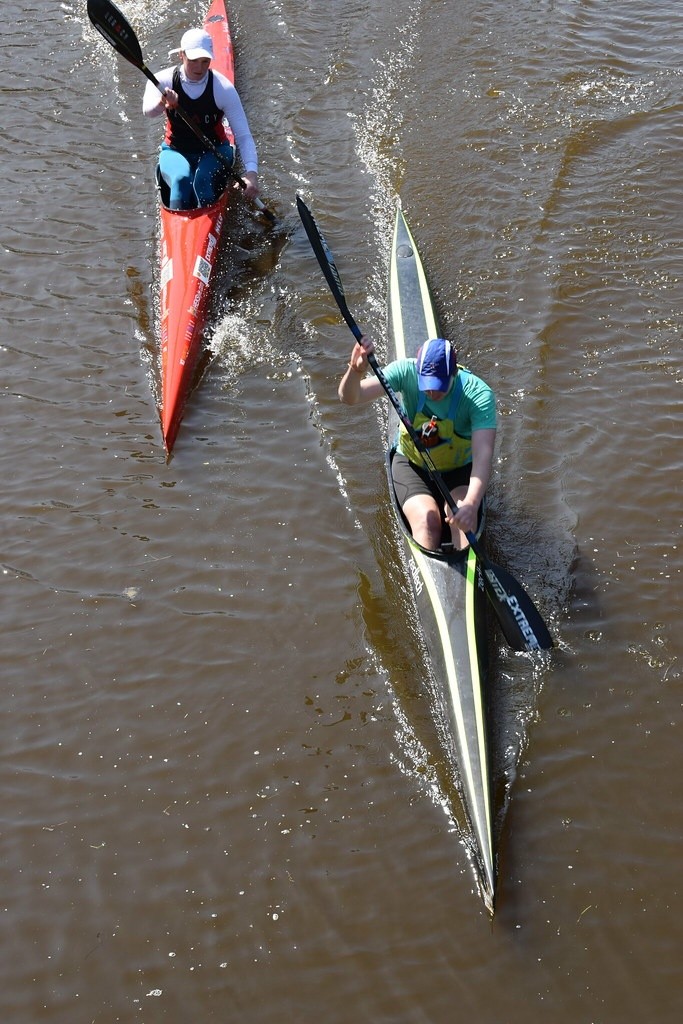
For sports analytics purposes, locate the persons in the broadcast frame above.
[338,335,497,551]
[142,27,259,211]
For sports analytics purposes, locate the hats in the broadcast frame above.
[180,27,215,61]
[415,338,456,392]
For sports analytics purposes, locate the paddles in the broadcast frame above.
[291,195,553,654]
[82,0,283,221]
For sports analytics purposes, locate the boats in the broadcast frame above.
[156,0,236,456]
[370,205,496,915]
